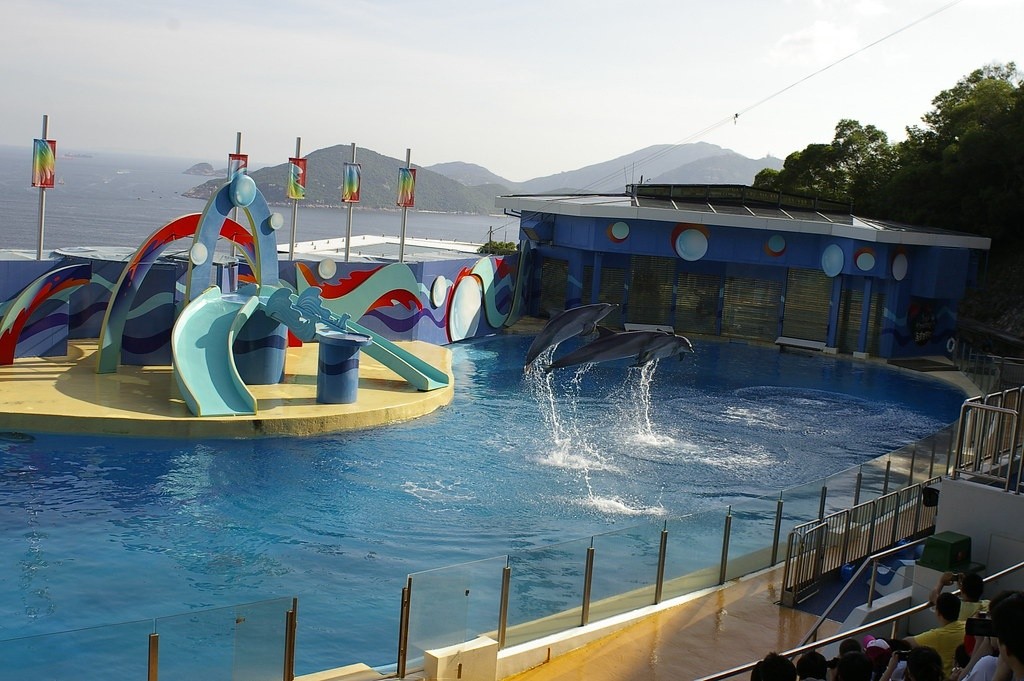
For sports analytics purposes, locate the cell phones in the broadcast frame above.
[827,658,840,668]
[895,651,910,661]
[965,612,997,636]
[950,574,959,581]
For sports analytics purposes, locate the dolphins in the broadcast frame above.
[540,325,695,372]
[523,302,620,376]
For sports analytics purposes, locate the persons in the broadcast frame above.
[989,590,1024,681]
[886,639,912,681]
[959,591,1021,681]
[930,571,991,621]
[863,634,892,681]
[750,661,764,681]
[902,592,967,681]
[834,651,872,681]
[796,651,828,681]
[879,645,943,681]
[761,651,797,681]
[947,643,970,681]
[830,639,861,681]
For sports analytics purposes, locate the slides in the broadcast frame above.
[171,286,260,417]
[255,286,449,391]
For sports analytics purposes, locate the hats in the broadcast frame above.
[863,634,892,663]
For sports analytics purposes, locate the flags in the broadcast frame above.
[287,157,307,199]
[396,168,416,207]
[31,139,56,188]
[341,161,361,203]
[228,154,248,182]
